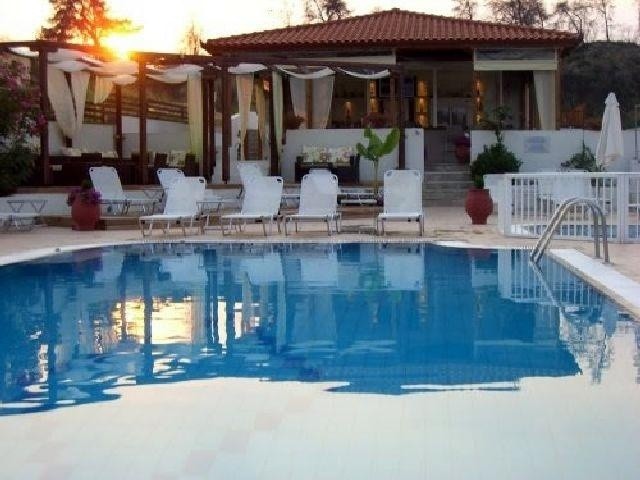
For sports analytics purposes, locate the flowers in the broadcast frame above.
[66,183,102,207]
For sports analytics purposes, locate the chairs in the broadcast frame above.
[138,175,206,236]
[309,167,378,211]
[283,173,341,236]
[88,166,158,215]
[237,162,299,208]
[155,167,236,211]
[221,176,285,237]
[375,169,425,237]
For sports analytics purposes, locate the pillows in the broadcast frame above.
[303,144,328,162]
[328,145,357,161]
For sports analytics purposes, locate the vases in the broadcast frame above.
[70,195,102,231]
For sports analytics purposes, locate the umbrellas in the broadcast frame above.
[596,91,623,167]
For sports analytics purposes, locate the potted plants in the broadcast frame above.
[465,172,494,225]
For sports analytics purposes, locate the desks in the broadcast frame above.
[197,197,235,232]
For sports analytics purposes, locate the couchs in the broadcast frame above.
[293,151,361,186]
